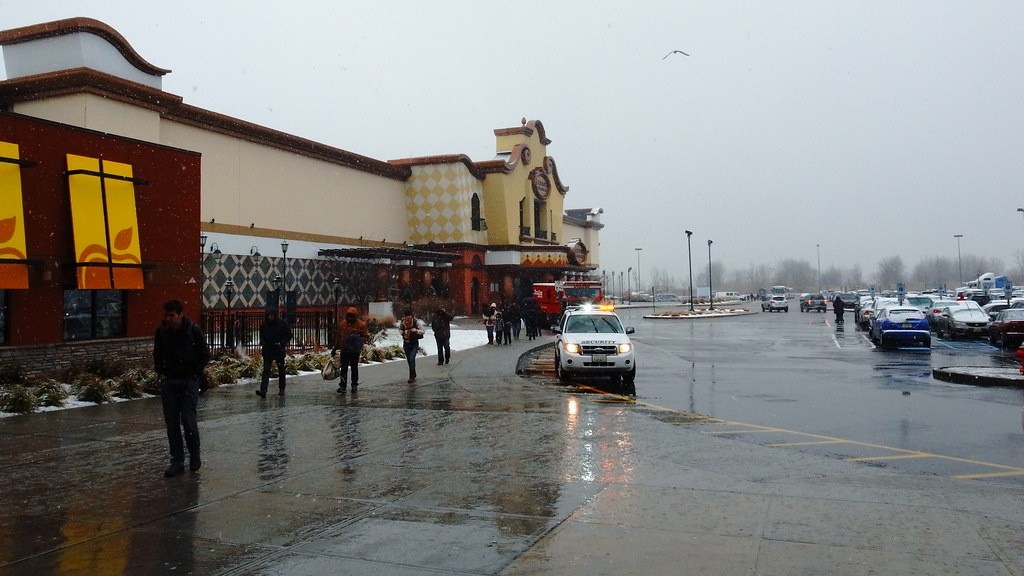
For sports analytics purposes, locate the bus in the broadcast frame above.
[770,286,795,299]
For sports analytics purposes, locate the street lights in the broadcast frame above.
[273,273,282,321]
[954,234,964,288]
[708,240,714,309]
[280,240,289,307]
[685,230,696,312]
[407,240,414,319]
[601,270,624,304]
[817,244,820,294]
[225,279,235,347]
[200,230,207,309]
[627,267,632,306]
[635,248,643,291]
[333,274,342,332]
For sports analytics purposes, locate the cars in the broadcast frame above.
[554,304,636,382]
[832,286,1024,352]
[800,293,827,313]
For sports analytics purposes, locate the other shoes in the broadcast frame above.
[351,386,357,392]
[278,390,285,395]
[336,386,347,393]
[190,446,200,472]
[446,359,449,364]
[408,376,415,382]
[529,336,535,341]
[437,362,443,365]
[517,336,519,340]
[509,339,511,344]
[514,336,517,340]
[490,342,493,345]
[497,342,502,345]
[165,458,185,475]
[503,342,507,345]
[256,388,266,399]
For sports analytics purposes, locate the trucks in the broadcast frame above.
[717,292,738,297]
[966,273,1014,288]
[535,280,604,327]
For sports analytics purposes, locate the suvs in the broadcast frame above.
[762,295,788,312]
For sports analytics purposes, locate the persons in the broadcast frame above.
[482,300,543,345]
[152,300,211,477]
[398,310,425,383]
[431,305,454,364]
[747,293,753,300]
[332,307,369,394]
[833,297,844,321]
[255,308,293,398]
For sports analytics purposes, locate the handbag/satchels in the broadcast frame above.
[410,333,423,340]
[321,357,341,380]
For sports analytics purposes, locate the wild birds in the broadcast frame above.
[662,50,689,59]
